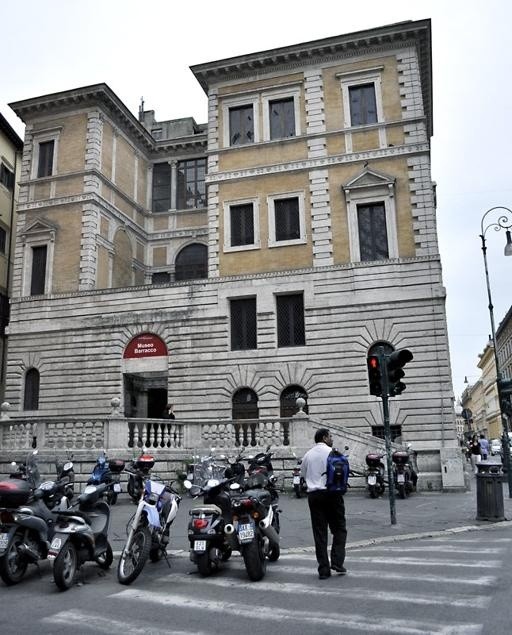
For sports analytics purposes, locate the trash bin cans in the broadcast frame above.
[474,463,505,521]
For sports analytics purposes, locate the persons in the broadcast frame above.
[299,426,347,581]
[469,435,482,478]
[479,433,489,461]
[163,404,175,419]
[465,435,473,460]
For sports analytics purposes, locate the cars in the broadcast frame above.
[491,437,502,454]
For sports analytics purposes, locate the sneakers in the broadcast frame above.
[331,563,346,571]
[320,572,332,579]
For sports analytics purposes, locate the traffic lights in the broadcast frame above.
[368,346,413,396]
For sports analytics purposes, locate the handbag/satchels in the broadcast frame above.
[323,448,350,495]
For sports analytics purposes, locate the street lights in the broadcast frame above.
[481,206,512,498]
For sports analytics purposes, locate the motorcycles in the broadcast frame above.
[0,448,124,590]
[366,441,418,498]
[118,468,182,584]
[126,451,155,505]
[183,446,282,581]
[292,453,307,497]
